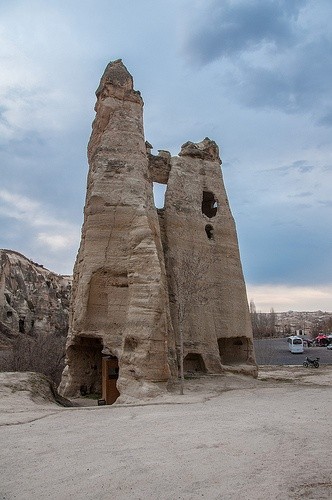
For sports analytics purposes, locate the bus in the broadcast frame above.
[287,336,303,353]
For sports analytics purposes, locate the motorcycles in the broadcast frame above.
[303,356,320,368]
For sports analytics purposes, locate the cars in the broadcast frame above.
[327,344,332,350]
